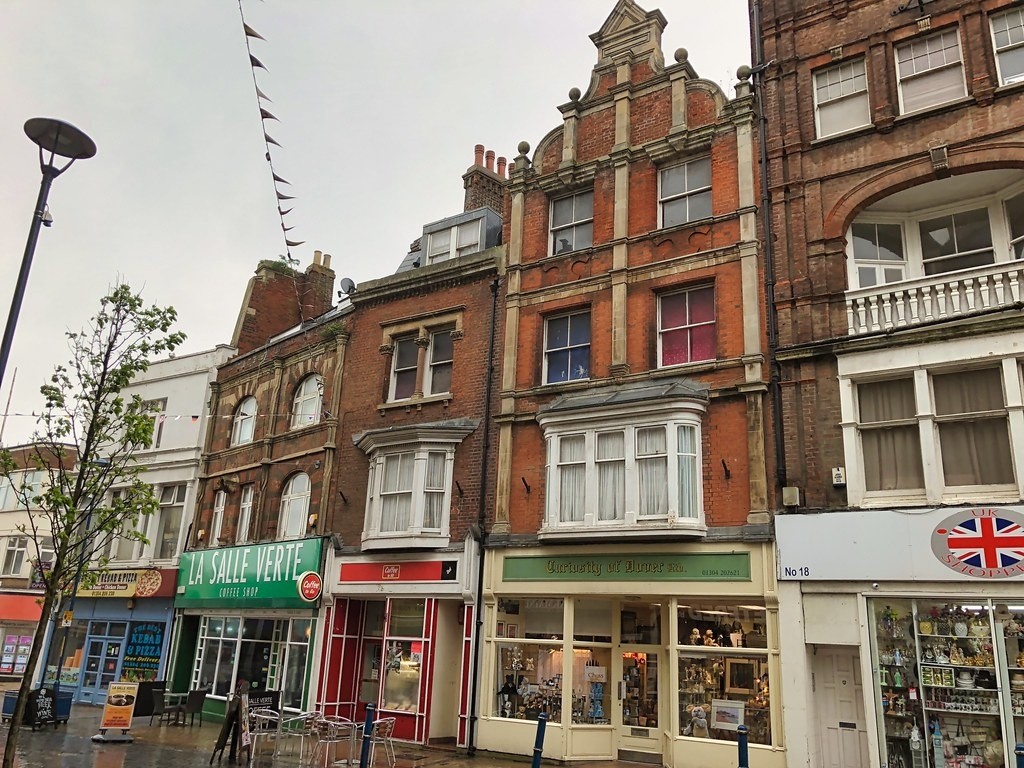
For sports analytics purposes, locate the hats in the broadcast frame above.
[956,669,996,690]
[993,604,1014,620]
[1010,673,1024,691]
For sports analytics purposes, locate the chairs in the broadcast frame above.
[250,708,397,768]
[149,689,206,728]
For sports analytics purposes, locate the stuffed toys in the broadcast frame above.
[704,630,717,646]
[683,704,711,738]
[749,672,769,709]
[587,682,605,717]
[690,628,701,644]
[679,661,725,692]
[497,657,552,720]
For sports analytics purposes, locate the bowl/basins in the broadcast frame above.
[972,625,991,636]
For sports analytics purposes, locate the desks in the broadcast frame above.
[162,693,188,726]
[258,715,293,755]
[334,722,365,764]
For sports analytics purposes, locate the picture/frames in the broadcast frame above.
[710,699,744,731]
[497,620,505,637]
[726,658,759,695]
[507,624,518,638]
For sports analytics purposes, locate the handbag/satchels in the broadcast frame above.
[952,719,970,755]
[942,734,953,758]
[966,719,990,741]
[966,746,984,765]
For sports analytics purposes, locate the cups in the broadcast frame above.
[112,693,126,705]
[588,683,604,716]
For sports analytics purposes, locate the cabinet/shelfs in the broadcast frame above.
[877,634,1024,741]
[589,682,603,724]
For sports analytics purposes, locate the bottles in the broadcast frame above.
[920,621,932,634]
[942,605,949,617]
[955,606,964,616]
[938,622,950,635]
[930,607,940,618]
[895,648,902,666]
[955,622,967,636]
[979,606,987,618]
